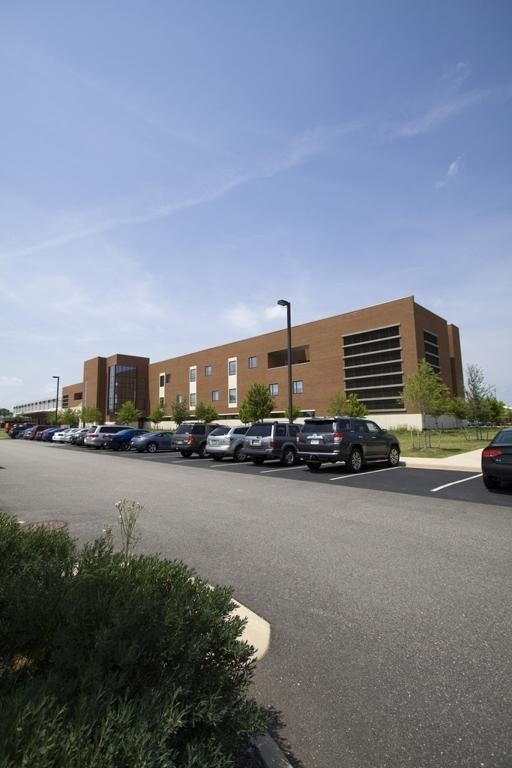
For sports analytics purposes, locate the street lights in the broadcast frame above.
[53,376,59,421]
[277,299,292,422]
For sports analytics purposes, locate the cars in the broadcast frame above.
[8,423,179,452]
[481,428,512,489]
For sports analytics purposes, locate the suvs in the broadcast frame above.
[297,417,401,473]
[171,421,305,466]
